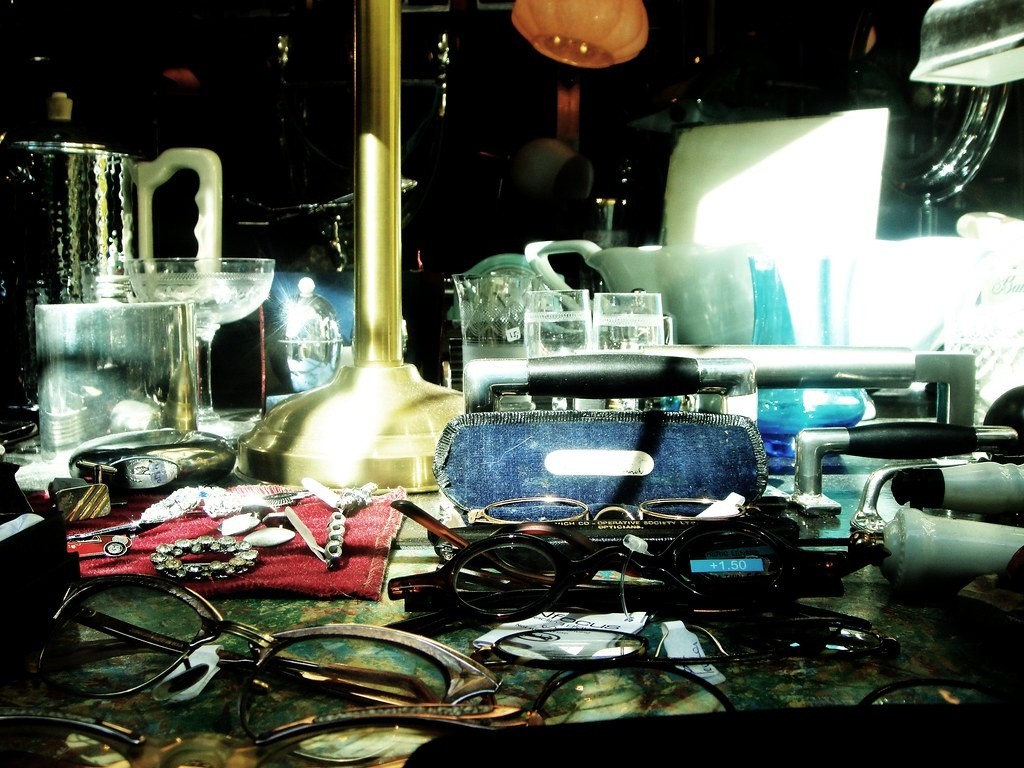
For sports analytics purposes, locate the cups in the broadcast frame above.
[452,270,544,415]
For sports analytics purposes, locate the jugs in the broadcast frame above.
[1,141,225,450]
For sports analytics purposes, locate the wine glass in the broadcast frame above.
[592,293,673,410]
[125,258,276,441]
[524,289,592,409]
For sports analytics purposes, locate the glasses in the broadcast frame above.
[482,520,886,602]
[471,611,904,669]
[0,696,542,768]
[30,572,500,763]
[525,657,1001,728]
[385,498,795,619]
[465,497,748,527]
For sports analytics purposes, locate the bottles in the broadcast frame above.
[579,197,630,295]
[35,274,198,452]
